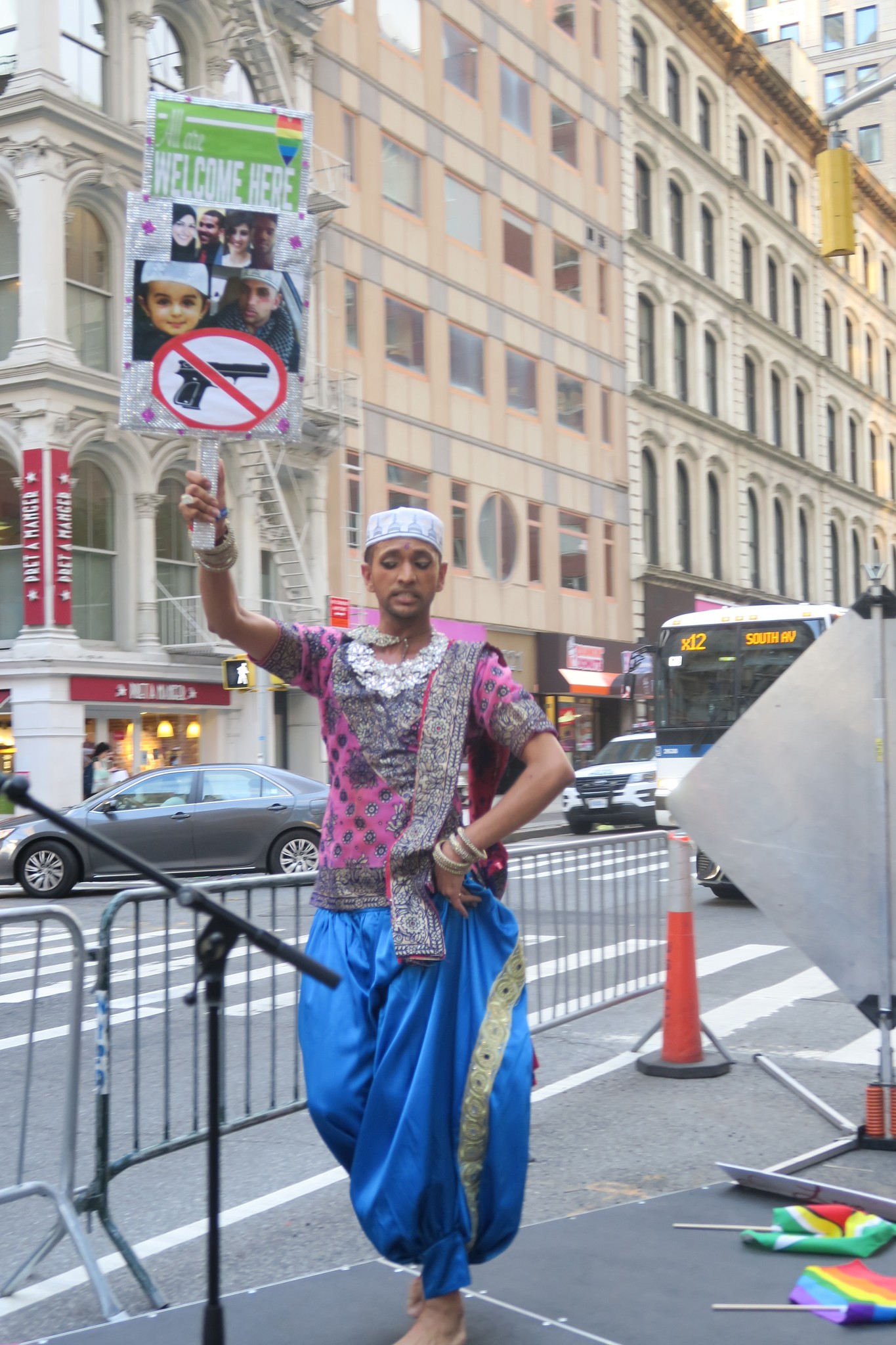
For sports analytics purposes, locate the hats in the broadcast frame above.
[140,260,209,296]
[365,506,444,561]
[240,269,284,290]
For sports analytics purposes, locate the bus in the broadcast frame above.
[621,603,850,900]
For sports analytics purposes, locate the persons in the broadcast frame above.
[83,742,112,800]
[132,204,300,372]
[179,460,575,1345]
[124,777,187,809]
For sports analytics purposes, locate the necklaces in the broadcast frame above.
[346,622,449,698]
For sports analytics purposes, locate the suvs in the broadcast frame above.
[561,720,670,835]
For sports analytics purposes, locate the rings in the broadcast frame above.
[181,494,193,505]
[448,893,461,902]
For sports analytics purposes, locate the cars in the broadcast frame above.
[0,763,331,899]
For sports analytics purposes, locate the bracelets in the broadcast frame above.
[449,833,480,864]
[186,507,228,532]
[187,518,239,572]
[432,840,472,875]
[458,827,488,860]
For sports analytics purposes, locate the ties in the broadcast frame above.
[199,249,207,263]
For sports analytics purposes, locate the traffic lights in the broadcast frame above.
[221,658,255,690]
